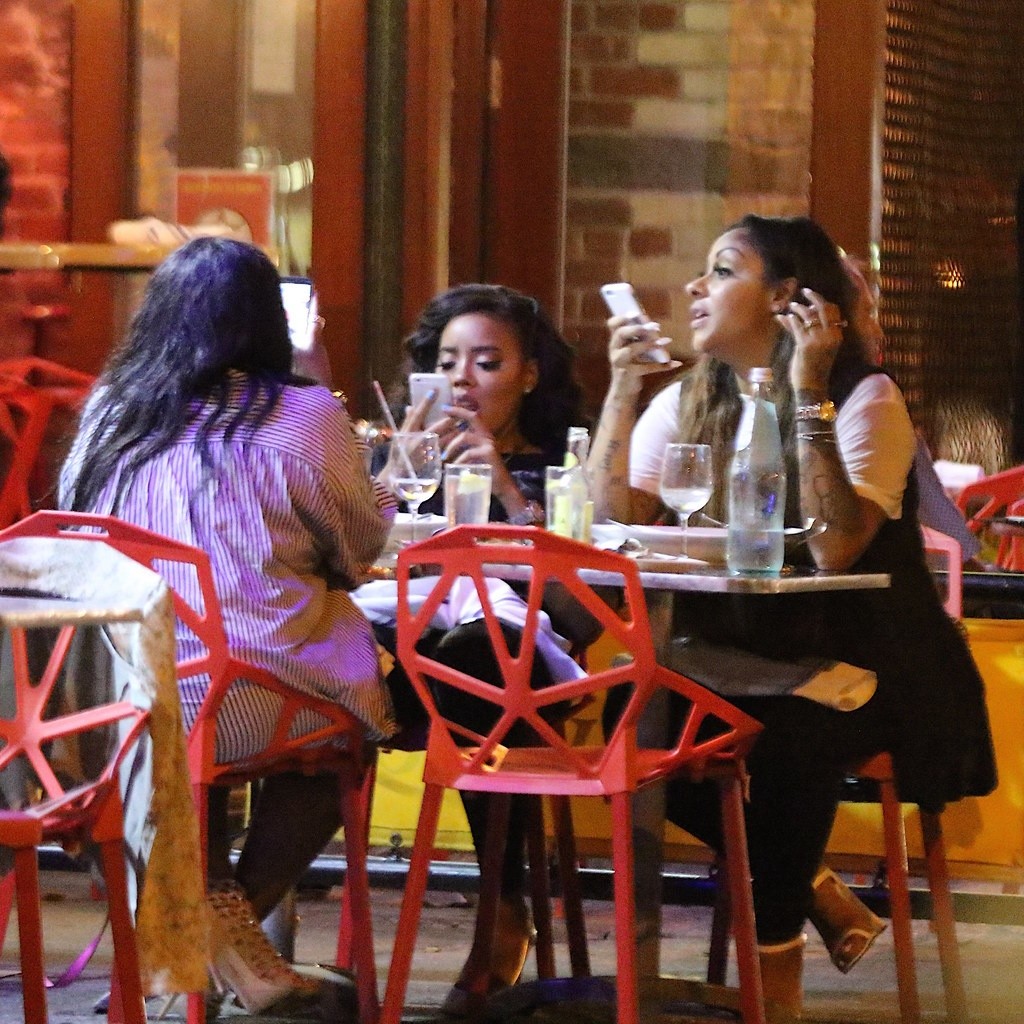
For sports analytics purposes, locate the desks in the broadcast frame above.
[0,242,280,276]
[362,557,890,1024]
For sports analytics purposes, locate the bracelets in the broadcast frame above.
[796,430,837,444]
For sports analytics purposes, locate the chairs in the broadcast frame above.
[710,524,967,1024]
[0,355,380,1024]
[381,522,770,1024]
[956,464,1024,571]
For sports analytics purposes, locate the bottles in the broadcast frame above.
[552,426,594,547]
[726,367,787,574]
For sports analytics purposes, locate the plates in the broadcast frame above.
[635,554,711,573]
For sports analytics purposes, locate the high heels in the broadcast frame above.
[197,874,357,1020]
[808,869,888,974]
[757,932,808,1024]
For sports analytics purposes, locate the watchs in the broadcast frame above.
[793,400,838,423]
[506,498,546,526]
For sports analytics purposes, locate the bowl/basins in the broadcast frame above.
[630,524,769,566]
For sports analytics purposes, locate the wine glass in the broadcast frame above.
[386,431,443,549]
[658,442,714,567]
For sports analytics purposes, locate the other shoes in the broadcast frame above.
[436,904,538,1017]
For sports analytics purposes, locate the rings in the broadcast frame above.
[829,319,848,327]
[804,318,820,330]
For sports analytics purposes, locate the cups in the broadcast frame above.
[543,466,569,533]
[443,462,493,527]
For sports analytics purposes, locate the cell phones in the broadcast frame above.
[409,373,453,431]
[600,283,669,363]
[277,276,317,350]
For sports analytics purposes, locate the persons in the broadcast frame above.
[363,274,623,1024]
[55,231,397,1024]
[584,208,998,1024]
